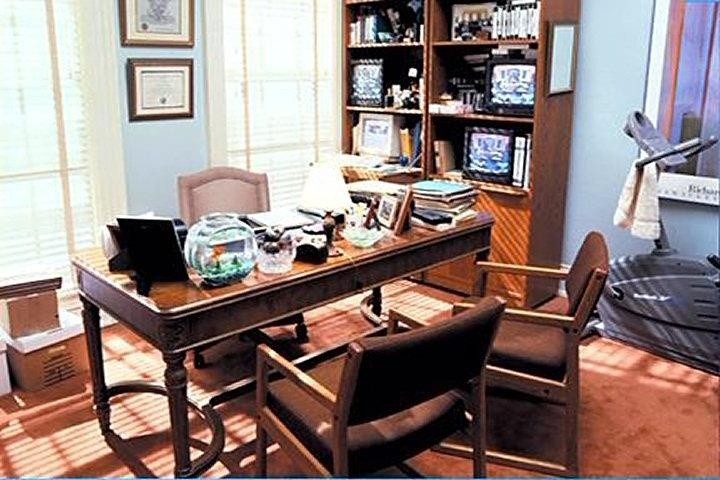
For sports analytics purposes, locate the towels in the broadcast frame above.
[612,155,661,240]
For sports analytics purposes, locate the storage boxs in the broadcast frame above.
[0,288,60,340]
[0,309,90,393]
[0,339,13,396]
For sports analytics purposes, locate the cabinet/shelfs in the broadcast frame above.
[333,0,580,309]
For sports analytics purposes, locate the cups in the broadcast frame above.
[254,233,296,274]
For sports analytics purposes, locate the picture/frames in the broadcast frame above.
[394,184,416,235]
[351,58,385,107]
[463,126,514,185]
[637,0,720,207]
[544,20,580,98]
[376,194,401,229]
[117,217,189,296]
[125,57,195,123]
[484,58,537,118]
[118,0,195,48]
[357,113,394,156]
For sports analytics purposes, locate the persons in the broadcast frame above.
[454,11,491,42]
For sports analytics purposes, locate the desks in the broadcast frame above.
[70,213,495,479]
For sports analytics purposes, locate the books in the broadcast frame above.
[348,14,394,44]
[246,180,481,233]
[491,2,540,42]
[433,135,532,190]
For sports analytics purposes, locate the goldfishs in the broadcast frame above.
[210,244,226,261]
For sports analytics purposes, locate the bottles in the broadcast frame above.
[455,7,494,41]
[384,88,394,110]
[323,208,336,247]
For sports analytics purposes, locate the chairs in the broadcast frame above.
[177,167,310,370]
[428,232,609,479]
[256,296,507,479]
